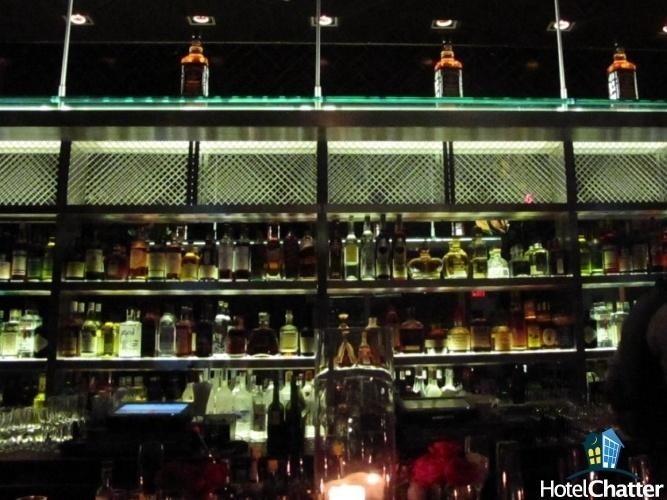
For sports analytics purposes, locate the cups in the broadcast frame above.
[309,324,398,499]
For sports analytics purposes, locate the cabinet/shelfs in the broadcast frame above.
[0,207,667,428]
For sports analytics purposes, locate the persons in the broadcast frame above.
[605,284,667,500]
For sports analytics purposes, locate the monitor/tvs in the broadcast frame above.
[110,402,192,417]
[399,396,473,413]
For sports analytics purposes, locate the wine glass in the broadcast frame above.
[0,396,96,464]
[476,371,618,432]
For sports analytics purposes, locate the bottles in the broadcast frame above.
[605,38,640,110]
[97,461,310,500]
[178,27,209,108]
[70,368,312,458]
[1,294,636,359]
[395,362,464,400]
[432,34,466,110]
[0,212,667,283]
